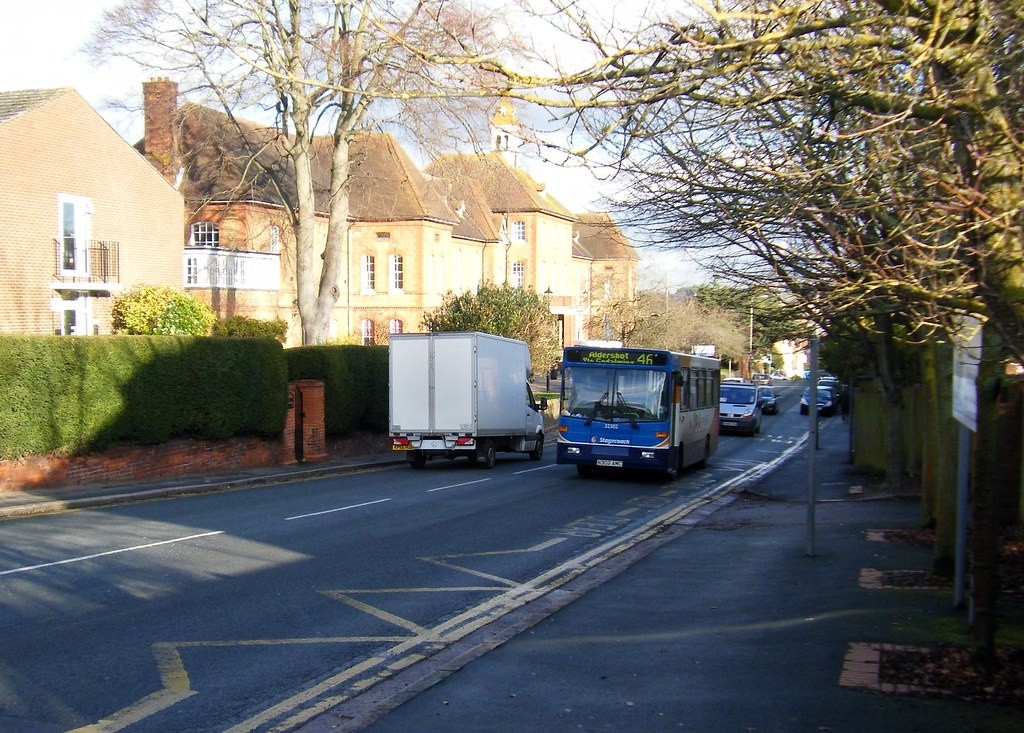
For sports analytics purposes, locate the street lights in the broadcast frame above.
[543,287,558,319]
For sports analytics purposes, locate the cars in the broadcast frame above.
[843,392,845,393]
[758,383,782,417]
[799,369,843,416]
[749,373,774,386]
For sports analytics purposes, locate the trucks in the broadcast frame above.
[388,330,547,470]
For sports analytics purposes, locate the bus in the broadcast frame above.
[555,345,723,485]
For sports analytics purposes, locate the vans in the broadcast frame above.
[719,381,764,436]
